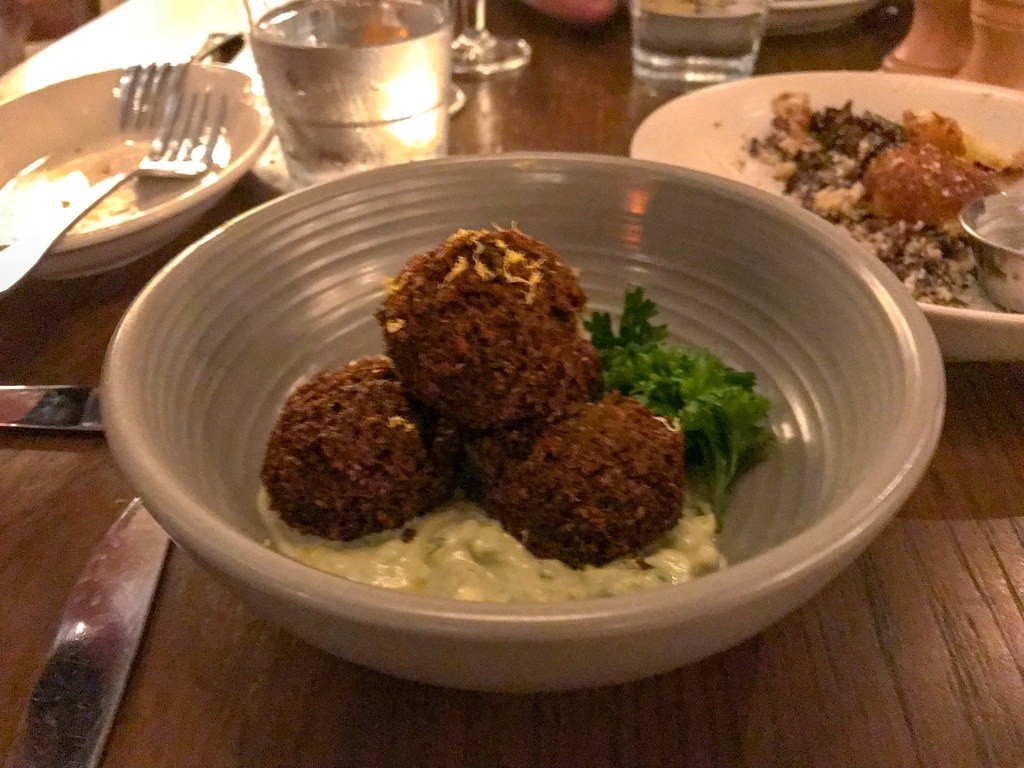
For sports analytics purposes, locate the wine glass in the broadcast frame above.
[451,0,532,75]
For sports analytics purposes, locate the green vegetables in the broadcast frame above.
[578,281,776,531]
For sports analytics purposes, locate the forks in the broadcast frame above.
[118,31,244,135]
[0,86,226,296]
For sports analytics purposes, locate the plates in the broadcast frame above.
[757,1,881,41]
[627,70,1024,367]
[0,64,275,279]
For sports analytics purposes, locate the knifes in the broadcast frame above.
[0,382,107,434]
[1,496,172,768]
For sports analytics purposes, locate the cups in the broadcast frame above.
[623,0,773,81]
[237,0,451,191]
[958,183,1024,311]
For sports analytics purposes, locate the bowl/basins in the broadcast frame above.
[99,153,948,693]
